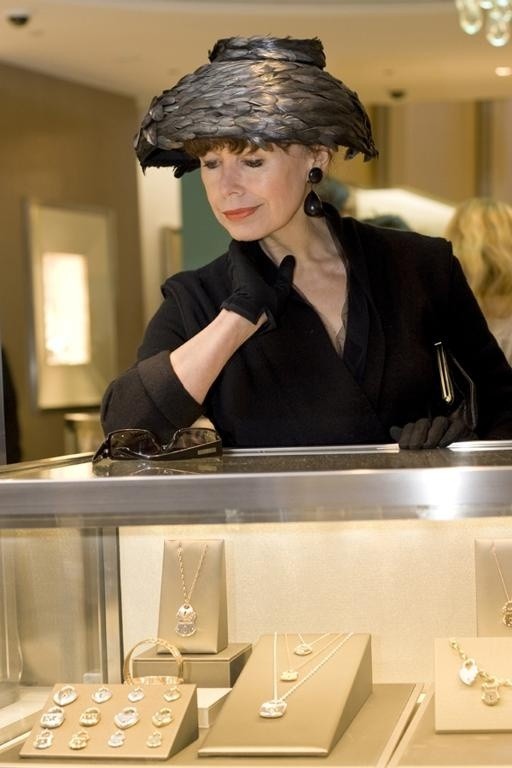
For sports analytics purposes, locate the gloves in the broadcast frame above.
[219,236,299,327]
[381,415,467,450]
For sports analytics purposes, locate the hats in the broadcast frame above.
[133,33,381,180]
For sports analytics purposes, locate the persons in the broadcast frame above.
[98,33,510,452]
[439,198,511,364]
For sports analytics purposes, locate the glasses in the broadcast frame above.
[80,424,229,469]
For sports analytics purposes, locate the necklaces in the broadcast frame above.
[258,629,355,719]
[291,631,334,657]
[176,541,209,639]
[447,635,512,707]
[490,540,512,627]
[278,631,347,682]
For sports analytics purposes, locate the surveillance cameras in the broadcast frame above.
[388,88,405,100]
[5,7,31,27]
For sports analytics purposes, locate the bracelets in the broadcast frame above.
[120,635,186,689]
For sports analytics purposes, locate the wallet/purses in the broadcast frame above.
[434,340,462,405]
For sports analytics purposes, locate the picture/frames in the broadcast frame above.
[20,194,126,417]
